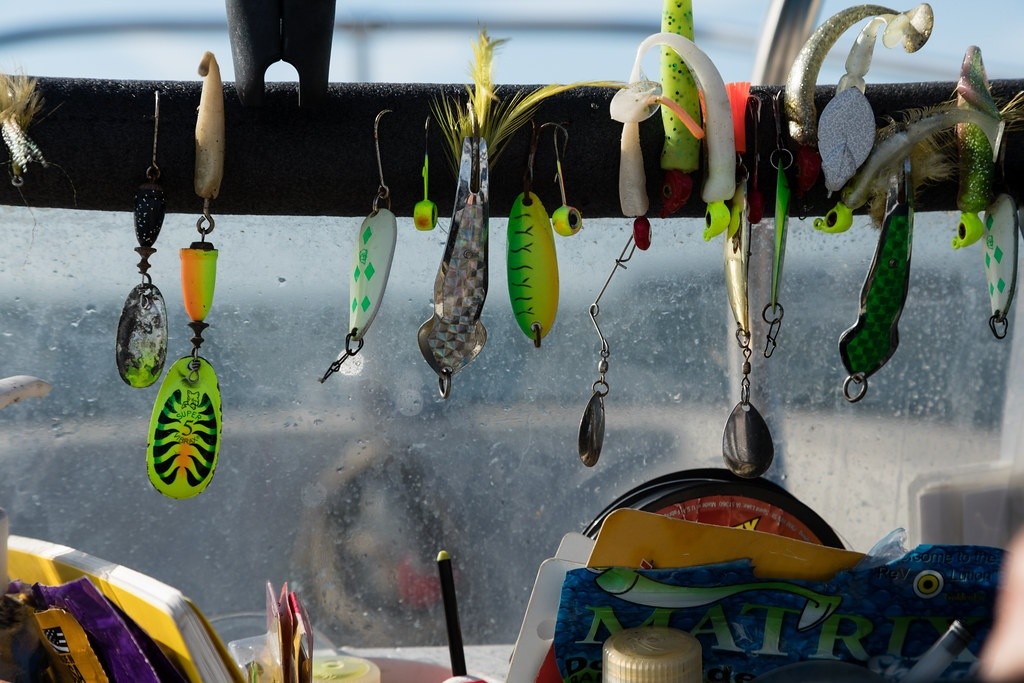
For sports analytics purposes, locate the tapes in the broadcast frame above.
[312,654,381,682]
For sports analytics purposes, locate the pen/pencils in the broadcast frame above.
[435,549,468,677]
[896,617,974,682]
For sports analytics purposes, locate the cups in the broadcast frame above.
[741,655,892,683]
